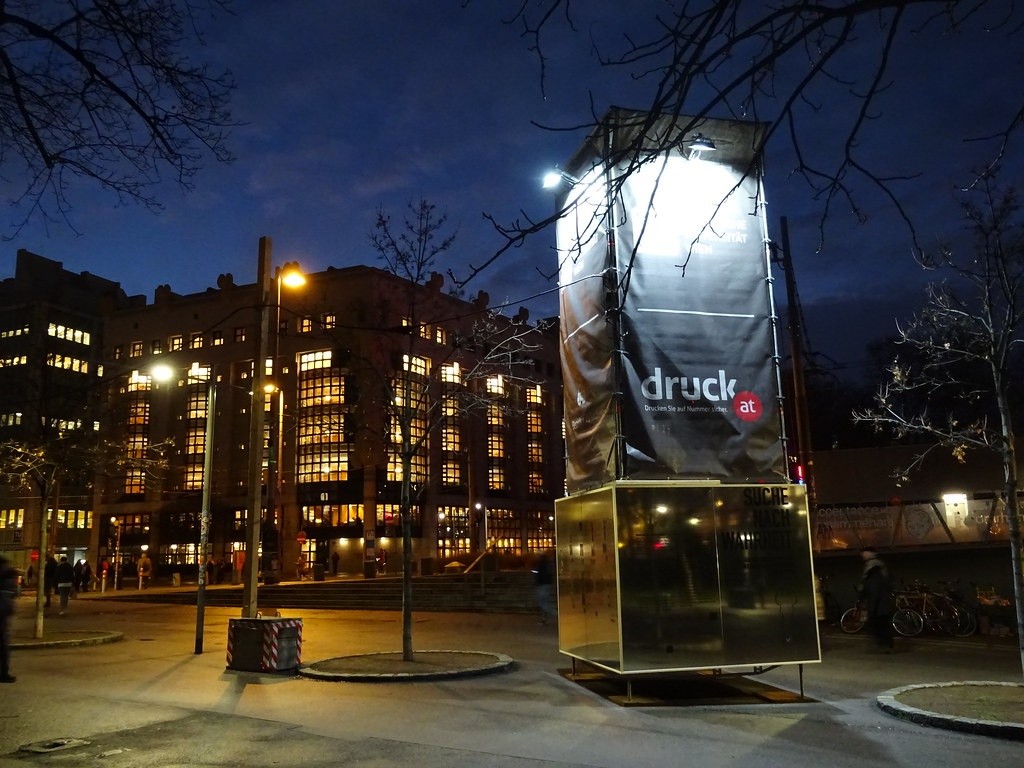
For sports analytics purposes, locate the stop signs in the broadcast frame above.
[30,552,39,561]
[297,532,306,544]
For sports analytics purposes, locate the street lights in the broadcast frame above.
[146,360,214,655]
[113,521,121,591]
[267,266,308,522]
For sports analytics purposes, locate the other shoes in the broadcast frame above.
[57,609,65,615]
[883,647,896,654]
[0,676,17,683]
[44,604,51,608]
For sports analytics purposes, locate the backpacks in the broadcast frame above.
[140,558,149,572]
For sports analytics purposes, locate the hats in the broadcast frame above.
[860,546,876,552]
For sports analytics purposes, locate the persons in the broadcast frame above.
[861,547,894,652]
[0,556,18,683]
[272,555,278,573]
[532,555,552,625]
[137,553,152,589]
[28,566,33,585]
[207,560,225,584]
[331,551,340,575]
[376,548,388,574]
[44,557,112,615]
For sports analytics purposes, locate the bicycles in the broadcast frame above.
[840,579,977,637]
